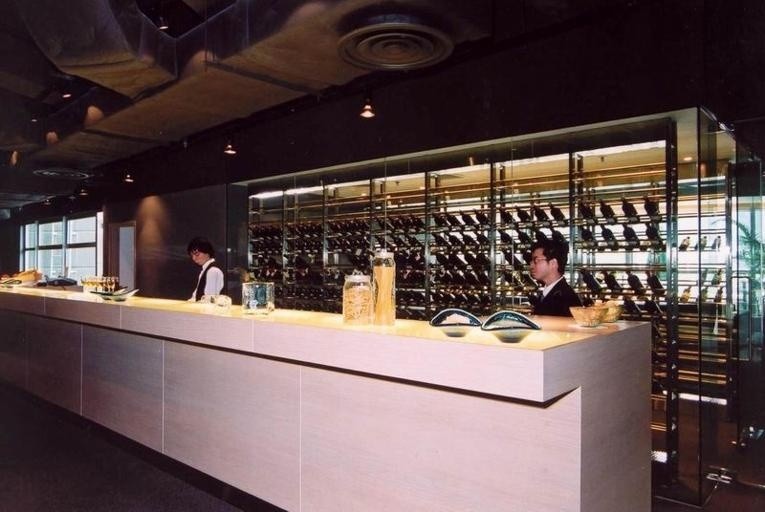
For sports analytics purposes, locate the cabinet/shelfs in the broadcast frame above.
[245,117,731,486]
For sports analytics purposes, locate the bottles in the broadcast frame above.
[250,192,723,313]
[342,251,397,327]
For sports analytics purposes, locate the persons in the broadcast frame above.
[185,238,228,303]
[525,240,582,318]
[257,257,281,279]
[293,255,310,280]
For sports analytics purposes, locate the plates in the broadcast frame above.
[89,287,139,301]
[429,306,542,345]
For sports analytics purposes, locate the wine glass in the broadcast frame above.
[79,274,119,295]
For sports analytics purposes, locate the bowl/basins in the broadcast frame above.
[568,305,624,326]
